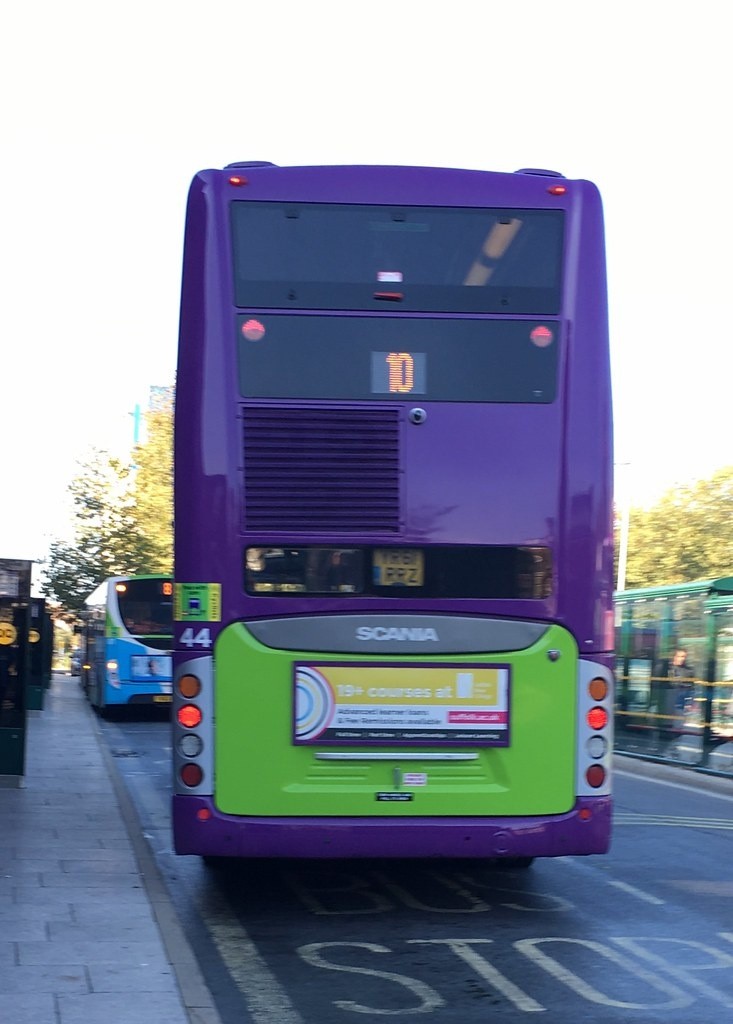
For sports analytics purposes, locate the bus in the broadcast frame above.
[170,158,622,874]
[74,574,175,718]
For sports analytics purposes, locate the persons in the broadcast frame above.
[667,650,695,734]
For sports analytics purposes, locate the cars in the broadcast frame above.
[68,650,82,676]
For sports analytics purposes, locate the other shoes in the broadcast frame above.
[653,749,664,755]
[671,747,680,755]
